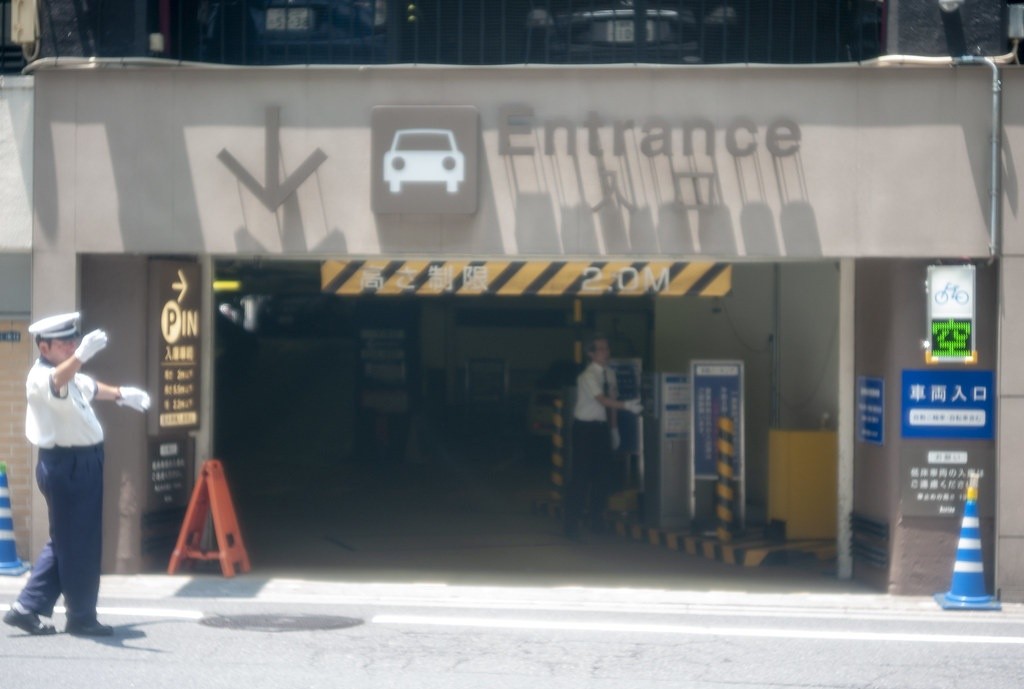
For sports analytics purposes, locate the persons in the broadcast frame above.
[573,334,643,533]
[2,311,152,636]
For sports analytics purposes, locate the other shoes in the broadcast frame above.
[564,524,613,544]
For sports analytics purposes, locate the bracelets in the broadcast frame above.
[115,385,121,401]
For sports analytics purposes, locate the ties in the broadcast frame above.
[603,370,613,422]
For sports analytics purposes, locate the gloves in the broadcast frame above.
[624,398,644,414]
[114,386,152,414]
[610,427,620,450]
[73,327,107,364]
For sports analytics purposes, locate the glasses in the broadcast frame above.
[596,347,608,351]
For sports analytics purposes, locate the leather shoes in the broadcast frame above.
[1,602,56,635]
[64,617,115,638]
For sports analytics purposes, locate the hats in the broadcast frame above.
[28,311,81,342]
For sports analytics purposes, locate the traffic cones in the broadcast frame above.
[0,457,32,574]
[933,480,1005,612]
